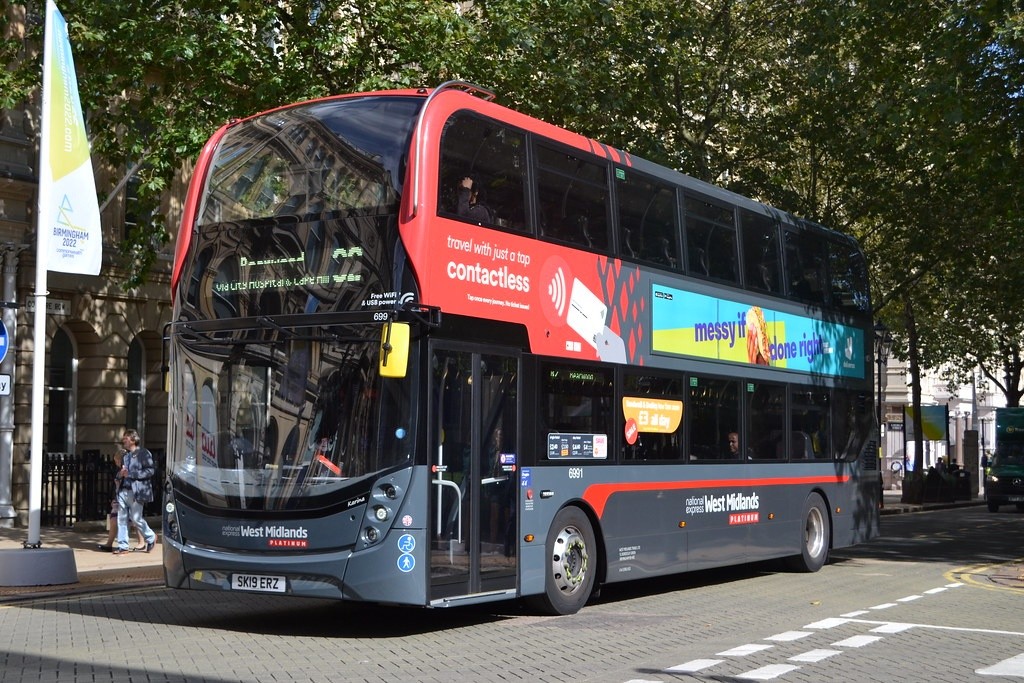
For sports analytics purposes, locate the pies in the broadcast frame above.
[746,307,770,362]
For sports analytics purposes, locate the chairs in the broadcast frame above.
[775,432,816,460]
[493,204,777,293]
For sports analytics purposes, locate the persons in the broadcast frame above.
[96,429,158,554]
[950,458,959,470]
[935,457,946,469]
[438,176,491,224]
[722,432,754,460]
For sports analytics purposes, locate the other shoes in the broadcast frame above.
[113,547,129,554]
[147,535,158,552]
[133,545,146,551]
[98,544,113,552]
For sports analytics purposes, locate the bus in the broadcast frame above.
[159,79,888,619]
[985,407,1024,513]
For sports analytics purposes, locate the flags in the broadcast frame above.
[46,0,103,275]
